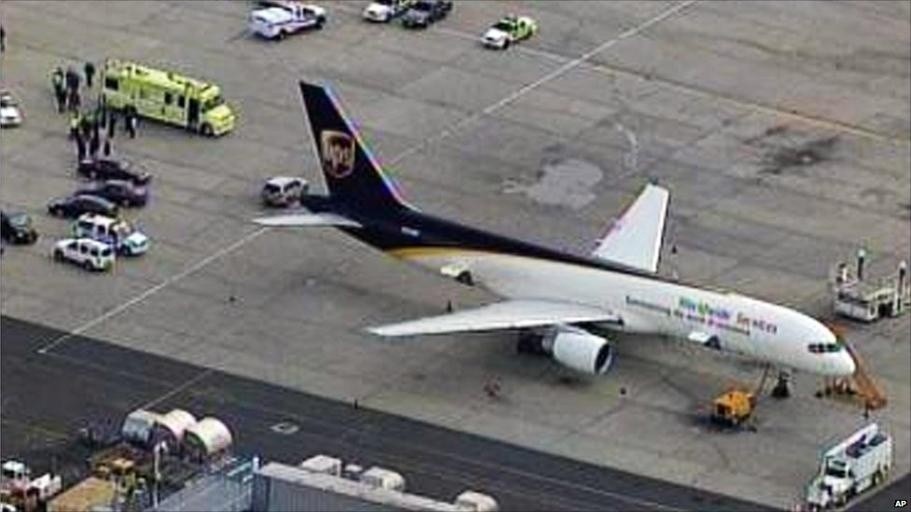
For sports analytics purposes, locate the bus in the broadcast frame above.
[98,56,235,138]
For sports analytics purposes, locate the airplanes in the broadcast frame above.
[249,79,858,378]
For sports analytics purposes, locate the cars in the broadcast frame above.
[1,208,40,248]
[1,93,24,128]
[360,1,420,23]
[400,1,452,30]
[478,14,540,50]
[246,0,328,41]
[45,156,155,275]
[260,176,309,210]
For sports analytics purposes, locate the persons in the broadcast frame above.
[831,261,854,297]
[49,58,139,160]
[0,24,6,53]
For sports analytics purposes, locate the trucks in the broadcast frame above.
[807,421,893,507]
